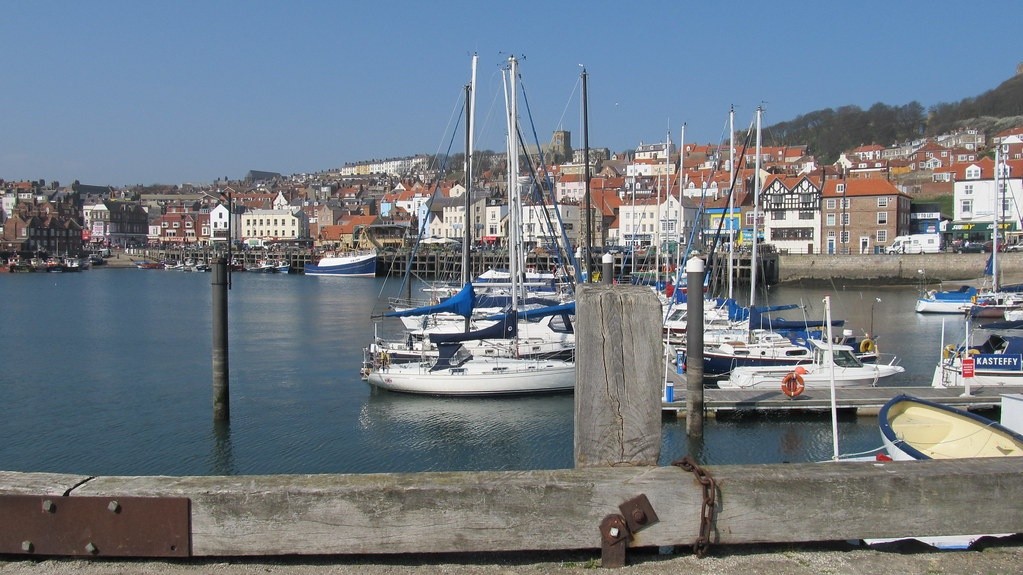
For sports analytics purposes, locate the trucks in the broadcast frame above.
[883,232,942,256]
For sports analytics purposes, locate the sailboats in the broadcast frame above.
[357,52,905,416]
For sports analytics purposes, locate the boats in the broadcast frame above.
[304,248,378,278]
[133,260,211,273]
[914,143,1023,392]
[258,260,291,275]
[10,236,80,273]
[877,392,1023,461]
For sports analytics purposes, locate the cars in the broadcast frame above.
[1007,242,1023,252]
[952,242,988,254]
[983,241,1007,253]
[88,254,103,265]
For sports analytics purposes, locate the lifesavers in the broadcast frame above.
[782,373,804,397]
[944,345,955,359]
[971,295,976,303]
[282,261,288,266]
[965,349,981,358]
[859,339,873,352]
[662,264,673,273]
[381,353,392,364]
